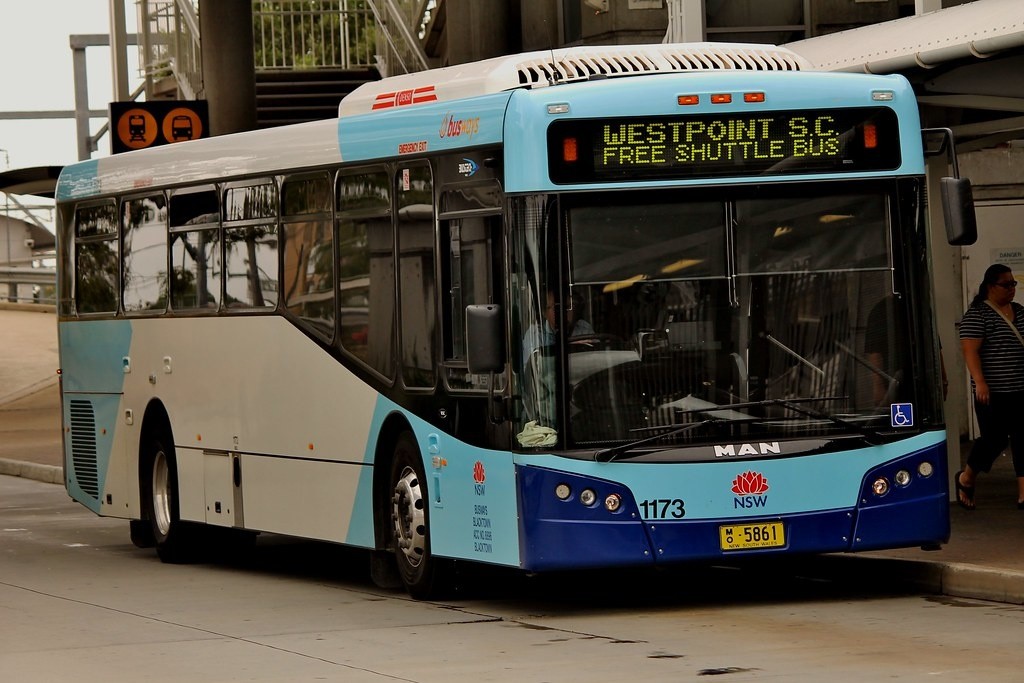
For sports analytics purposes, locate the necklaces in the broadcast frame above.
[992,303,1009,316]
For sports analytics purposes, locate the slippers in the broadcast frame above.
[1017,500,1024,510]
[955,471,976,511]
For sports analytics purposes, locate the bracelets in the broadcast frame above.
[944,378,948,386]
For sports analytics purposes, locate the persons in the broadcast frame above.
[863,275,948,422]
[955,265,1024,510]
[523,283,606,430]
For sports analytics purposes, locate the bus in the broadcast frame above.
[53,46,978,608]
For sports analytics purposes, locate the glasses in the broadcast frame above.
[994,280,1017,288]
